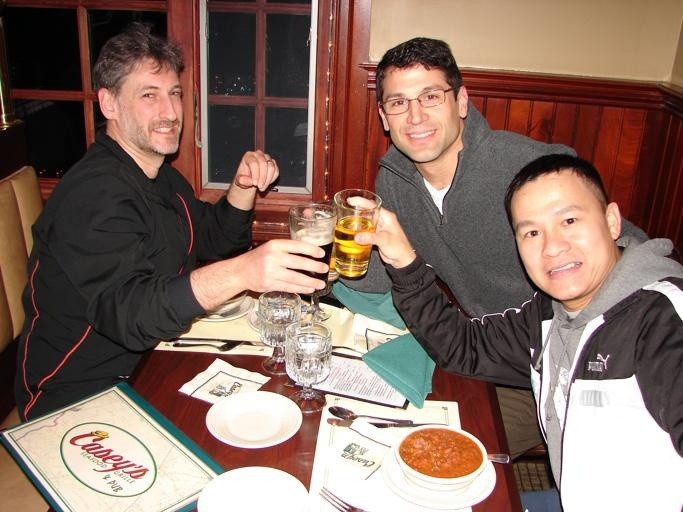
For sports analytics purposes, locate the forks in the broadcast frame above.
[318,486,366,512]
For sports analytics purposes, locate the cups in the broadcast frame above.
[289,189,382,285]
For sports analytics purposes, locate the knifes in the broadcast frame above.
[173,337,267,346]
[327,418,418,427]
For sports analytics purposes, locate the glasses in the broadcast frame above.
[379,86,459,115]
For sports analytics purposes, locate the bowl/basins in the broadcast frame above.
[395,425,488,491]
[209,290,248,314]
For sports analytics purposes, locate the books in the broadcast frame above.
[0,384,225,512]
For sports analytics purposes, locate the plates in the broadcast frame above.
[381,448,496,509]
[206,391,303,449]
[194,296,255,322]
[197,466,315,512]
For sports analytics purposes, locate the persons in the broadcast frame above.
[347,153,683,512]
[303,37,652,464]
[10,30,330,426]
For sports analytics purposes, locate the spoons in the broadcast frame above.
[173,341,244,351]
[328,406,413,425]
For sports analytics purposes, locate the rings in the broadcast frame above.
[265,160,272,162]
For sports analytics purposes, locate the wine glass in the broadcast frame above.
[258,282,333,413]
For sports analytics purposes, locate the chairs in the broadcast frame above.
[0,164,50,512]
